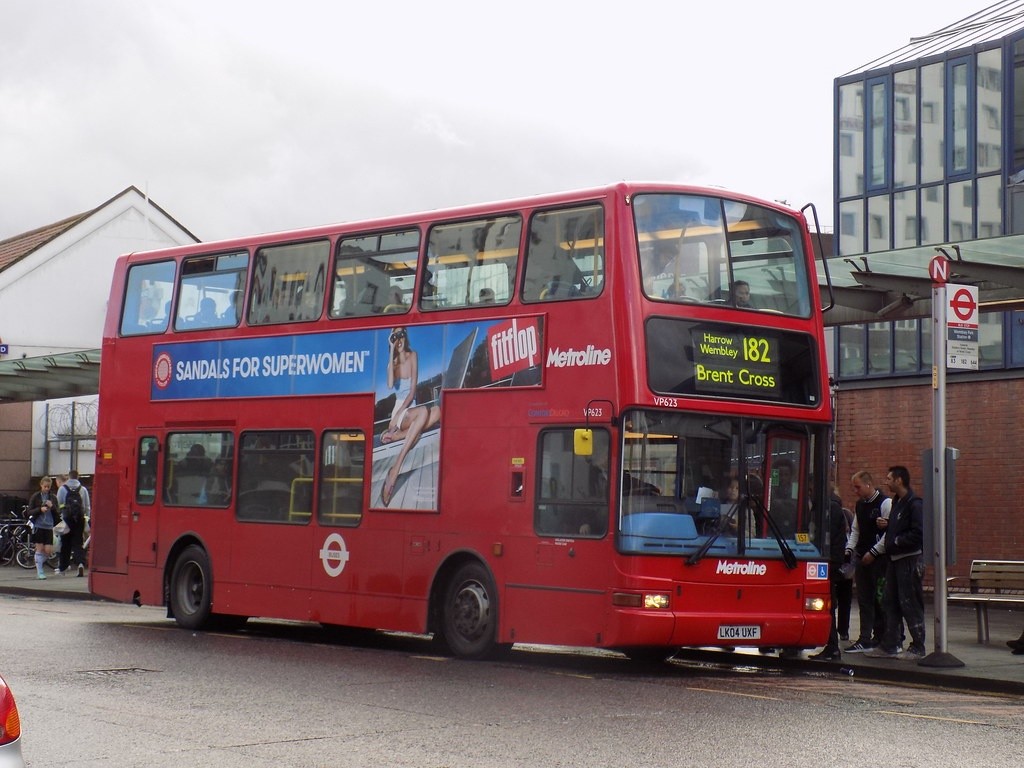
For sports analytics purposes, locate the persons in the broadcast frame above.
[723,479,756,538]
[380,326,441,508]
[479,288,495,303]
[381,285,407,312]
[565,441,659,536]
[195,297,216,323]
[54,470,91,578]
[668,283,687,299]
[735,280,750,307]
[225,290,245,325]
[175,444,232,504]
[808,474,854,661]
[844,471,903,653]
[162,300,183,324]
[27,476,60,580]
[265,286,318,322]
[863,466,926,657]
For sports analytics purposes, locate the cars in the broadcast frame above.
[0,676,24,768]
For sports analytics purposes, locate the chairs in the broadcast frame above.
[150,319,164,332]
[185,315,194,328]
[220,313,226,325]
[540,281,582,299]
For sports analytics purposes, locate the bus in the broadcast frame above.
[89,182,833,665]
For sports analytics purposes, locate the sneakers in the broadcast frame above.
[843,640,873,652]
[864,645,897,658]
[898,647,903,653]
[897,646,927,660]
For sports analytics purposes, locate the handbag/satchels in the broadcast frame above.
[53,517,70,535]
[27,516,37,536]
[876,574,893,609]
[839,564,855,579]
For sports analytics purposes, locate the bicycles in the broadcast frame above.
[0,505,60,570]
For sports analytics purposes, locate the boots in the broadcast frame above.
[34,554,47,580]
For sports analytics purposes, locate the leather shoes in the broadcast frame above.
[808,646,842,661]
[841,632,849,640]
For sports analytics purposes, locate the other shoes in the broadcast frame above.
[1006,632,1024,651]
[75,563,84,578]
[54,567,65,578]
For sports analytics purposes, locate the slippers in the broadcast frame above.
[381,467,394,507]
[380,429,394,444]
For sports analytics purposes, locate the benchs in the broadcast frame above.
[946,560,1024,644]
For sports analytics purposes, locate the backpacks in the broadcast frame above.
[63,485,83,523]
